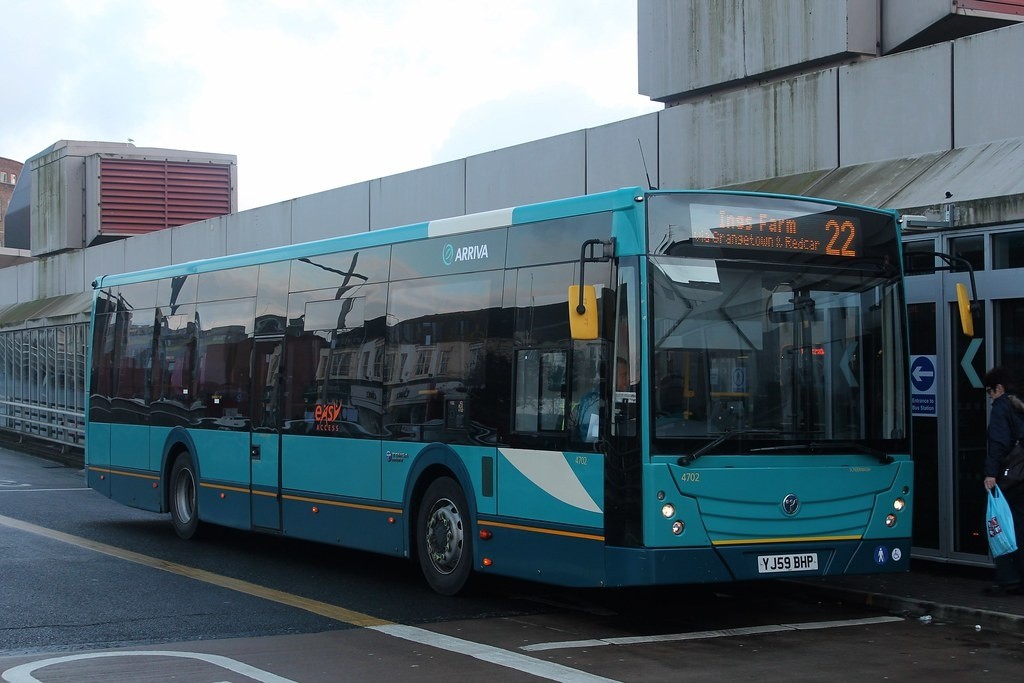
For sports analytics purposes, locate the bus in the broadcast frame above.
[84,187,981,599]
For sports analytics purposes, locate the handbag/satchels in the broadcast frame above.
[986,483,1018,558]
[988,396,1024,491]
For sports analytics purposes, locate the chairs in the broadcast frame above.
[213,384,244,411]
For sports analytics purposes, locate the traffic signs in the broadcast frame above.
[909,354,938,416]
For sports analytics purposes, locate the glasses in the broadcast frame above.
[987,388,991,394]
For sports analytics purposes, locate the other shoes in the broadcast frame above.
[982,582,1024,597]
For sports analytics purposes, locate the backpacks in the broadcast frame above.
[567,404,580,442]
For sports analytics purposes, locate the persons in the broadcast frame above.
[979,366,1024,598]
[577,356,638,444]
